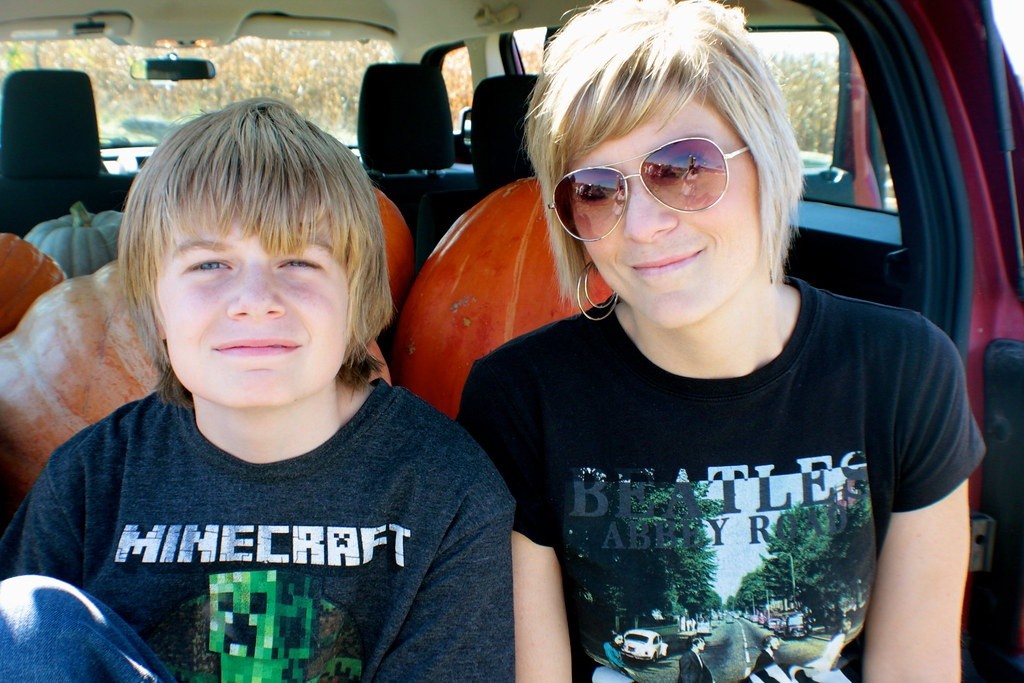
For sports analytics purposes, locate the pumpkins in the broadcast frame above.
[0,176,617,533]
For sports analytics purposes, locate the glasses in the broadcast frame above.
[548,138,750,241]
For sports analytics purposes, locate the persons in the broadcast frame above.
[457,2,986,683]
[556,173,627,237]
[644,154,702,211]
[0,98,515,683]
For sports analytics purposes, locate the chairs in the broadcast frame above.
[472,74,544,195]
[355,62,456,273]
[0,69,104,240]
